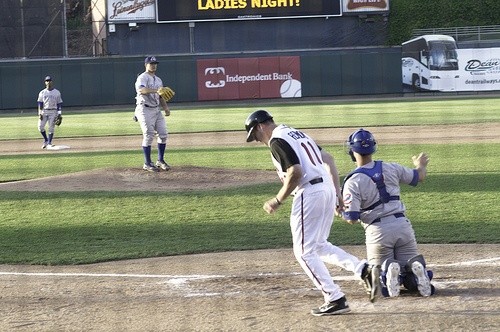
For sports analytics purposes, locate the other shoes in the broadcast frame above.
[42,141,48,149]
[48,143,55,148]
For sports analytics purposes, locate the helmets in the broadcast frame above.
[244,109,273,142]
[348,128,377,155]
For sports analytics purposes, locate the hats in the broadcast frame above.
[45,75,53,81]
[144,55,159,64]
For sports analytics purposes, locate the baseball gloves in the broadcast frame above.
[53,116,62,126]
[157,87,175,102]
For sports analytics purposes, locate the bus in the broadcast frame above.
[400,34,459,92]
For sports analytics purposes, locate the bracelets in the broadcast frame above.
[58,111,61,114]
[276,197,282,205]
[39,110,43,115]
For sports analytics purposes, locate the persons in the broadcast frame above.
[337,127,435,299]
[36,76,62,149]
[134,56,173,173]
[245,109,381,318]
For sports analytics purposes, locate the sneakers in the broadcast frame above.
[143,163,160,172]
[385,261,401,297]
[311,300,351,317]
[155,160,170,171]
[411,260,431,297]
[362,264,379,303]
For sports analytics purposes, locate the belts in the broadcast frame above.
[308,177,323,185]
[45,109,54,110]
[371,213,405,224]
[140,103,157,108]
